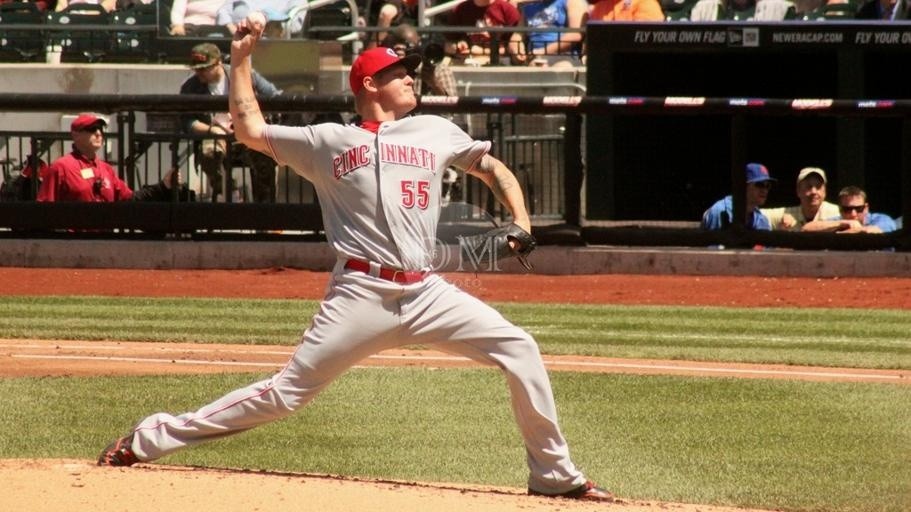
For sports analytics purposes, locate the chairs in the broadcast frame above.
[0,1,911,75]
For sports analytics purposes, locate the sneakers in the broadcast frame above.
[96,433,147,469]
[527,475,614,504]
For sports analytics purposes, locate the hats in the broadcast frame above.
[744,163,777,183]
[796,166,827,184]
[189,41,224,71]
[71,113,108,131]
[349,44,423,97]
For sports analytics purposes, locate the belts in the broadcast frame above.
[343,257,433,288]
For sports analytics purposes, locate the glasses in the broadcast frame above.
[840,204,865,213]
[750,181,772,189]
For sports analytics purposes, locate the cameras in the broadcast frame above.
[401,36,449,67]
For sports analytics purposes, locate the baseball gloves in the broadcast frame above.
[457,223,536,266]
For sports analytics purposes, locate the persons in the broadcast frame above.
[34,113,182,232]
[384,24,460,120]
[0,1,670,68]
[771,167,842,232]
[175,43,285,204]
[798,185,897,252]
[97,15,614,502]
[697,163,780,248]
[857,0,911,20]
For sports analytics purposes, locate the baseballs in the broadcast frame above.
[245,12,266,32]
